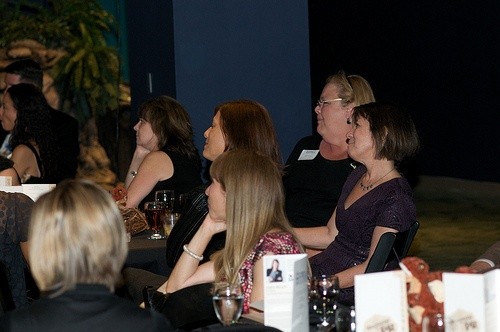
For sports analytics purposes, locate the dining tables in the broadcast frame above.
[118,227,170,265]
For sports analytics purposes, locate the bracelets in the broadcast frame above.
[182,243,204,261]
[128,168,137,179]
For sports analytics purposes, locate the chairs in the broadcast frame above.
[336,221,419,305]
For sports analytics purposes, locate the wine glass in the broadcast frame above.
[311,276,339,326]
[143,201,168,239]
[155,190,176,239]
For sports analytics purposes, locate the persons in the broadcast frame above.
[265,258,282,282]
[0,57,500,332]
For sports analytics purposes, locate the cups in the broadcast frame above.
[211,282,246,327]
[336,307,356,332]
[0,176,12,186]
[163,215,180,238]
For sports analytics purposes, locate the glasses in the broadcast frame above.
[317,97,345,109]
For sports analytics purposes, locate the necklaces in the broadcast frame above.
[358,168,395,192]
